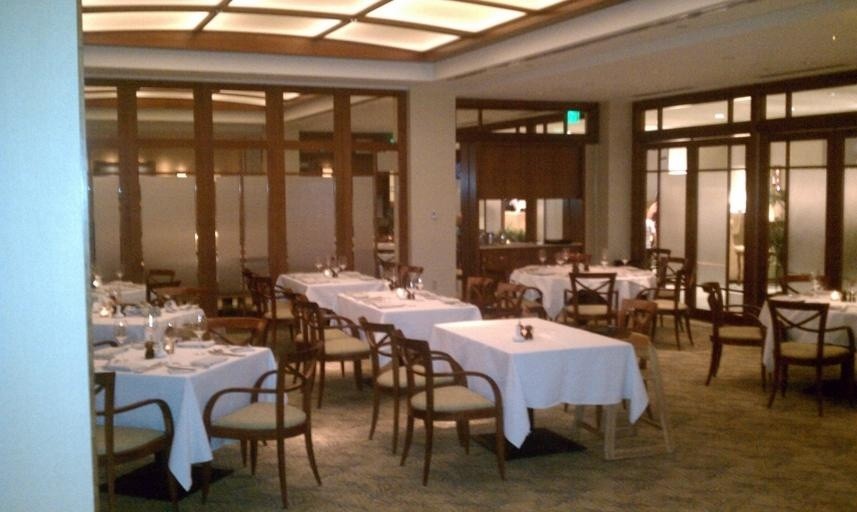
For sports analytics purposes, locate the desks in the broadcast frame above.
[768,293,855,398]
[426,312,647,461]
[89,341,283,488]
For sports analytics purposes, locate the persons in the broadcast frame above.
[645,213,656,262]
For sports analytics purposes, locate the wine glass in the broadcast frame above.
[804,268,856,306]
[381,263,424,304]
[537,247,632,275]
[310,251,351,281]
[109,309,212,368]
[90,267,176,319]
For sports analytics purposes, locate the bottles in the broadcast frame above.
[512,322,534,342]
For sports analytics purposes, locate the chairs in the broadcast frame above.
[198,338,326,504]
[765,297,856,417]
[85,248,695,349]
[779,270,827,297]
[701,280,765,386]
[592,297,673,459]
[90,368,177,510]
[391,328,511,486]
[297,298,372,409]
[356,314,466,444]
